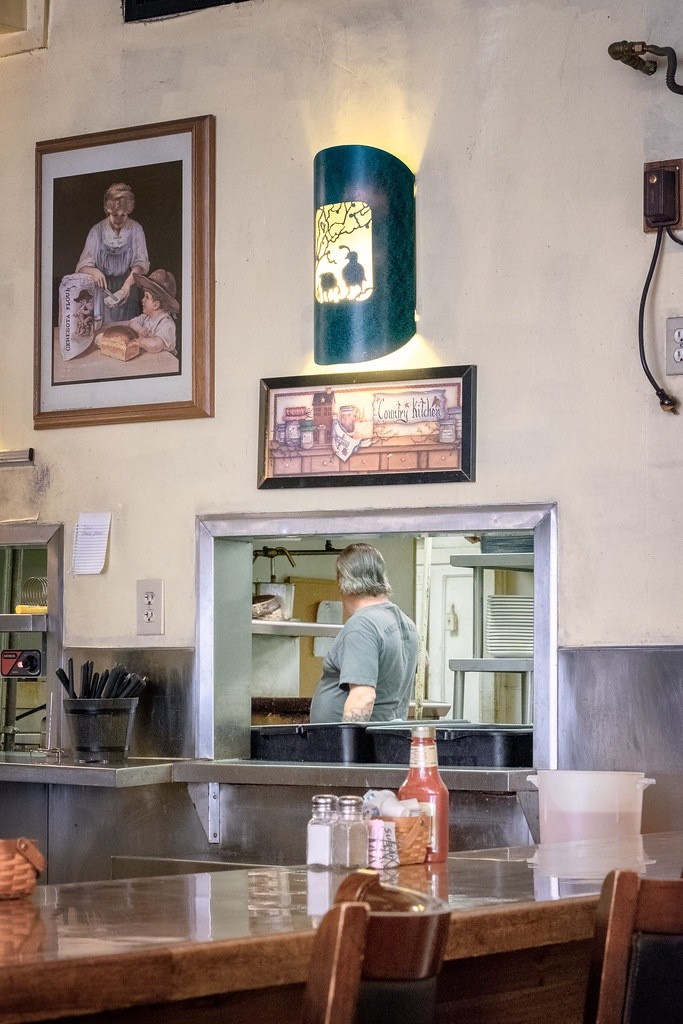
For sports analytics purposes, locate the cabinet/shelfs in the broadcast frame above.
[449,546,538,731]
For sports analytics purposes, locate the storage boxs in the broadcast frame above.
[368,713,532,766]
[250,718,386,766]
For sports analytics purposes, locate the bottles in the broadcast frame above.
[306,794,338,867]
[398,726,449,863]
[337,796,369,868]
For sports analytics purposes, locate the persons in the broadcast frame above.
[310,541,420,725]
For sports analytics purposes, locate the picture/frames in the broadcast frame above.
[32,113,220,429]
[122,0,244,25]
[256,366,477,488]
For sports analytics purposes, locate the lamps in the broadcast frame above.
[312,143,417,365]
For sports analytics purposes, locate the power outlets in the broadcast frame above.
[136,578,166,634]
[666,318,683,376]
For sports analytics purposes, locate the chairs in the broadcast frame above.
[584,869,683,1024]
[300,872,454,1023]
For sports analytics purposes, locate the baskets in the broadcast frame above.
[0,838,45,900]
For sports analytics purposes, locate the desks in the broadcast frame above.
[0,825,683,1023]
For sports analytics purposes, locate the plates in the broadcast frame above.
[485,594,534,657]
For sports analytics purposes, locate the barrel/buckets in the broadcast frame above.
[63,698,139,765]
[526,769,656,844]
